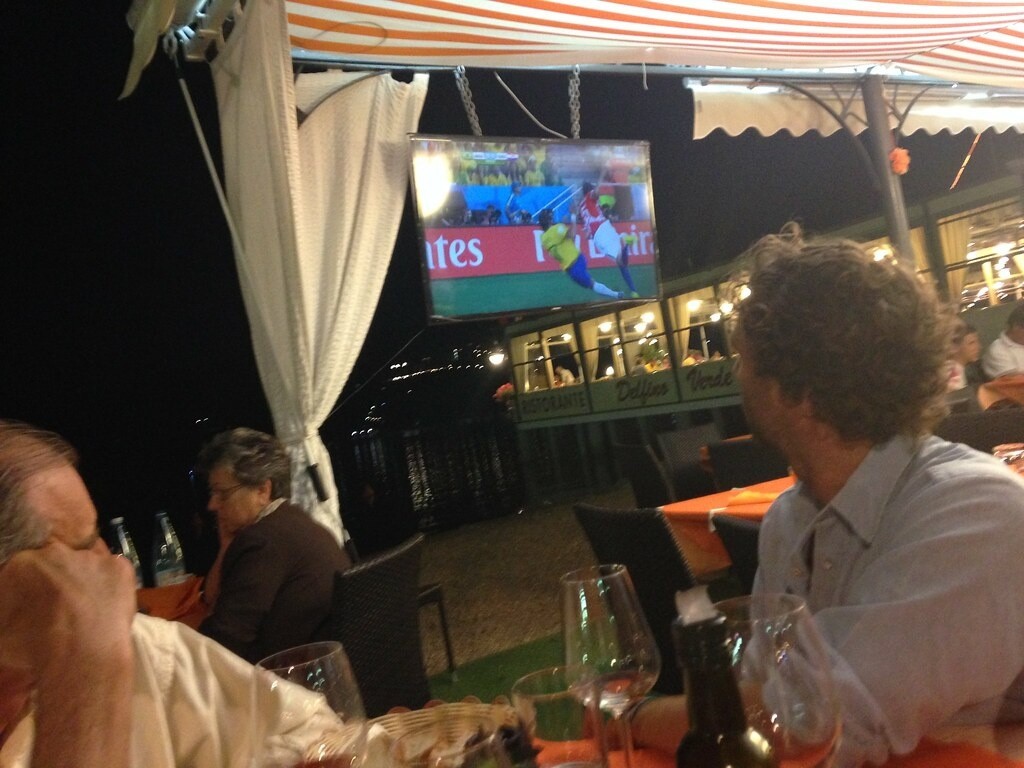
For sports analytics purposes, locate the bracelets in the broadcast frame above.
[622,697,654,749]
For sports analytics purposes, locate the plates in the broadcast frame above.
[297,697,537,768]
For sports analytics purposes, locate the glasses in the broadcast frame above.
[209,479,245,500]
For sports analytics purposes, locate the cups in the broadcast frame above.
[710,593,843,768]
[511,664,604,768]
[389,715,510,768]
[252,641,367,768]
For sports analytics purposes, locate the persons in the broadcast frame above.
[554,349,723,387]
[192,427,341,666]
[539,208,624,299]
[414,141,647,228]
[980,304,1024,380]
[595,222,1023,768]
[0,420,345,767]
[577,182,640,298]
[937,327,983,393]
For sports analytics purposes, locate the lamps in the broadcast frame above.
[488,342,505,364]
[561,285,754,343]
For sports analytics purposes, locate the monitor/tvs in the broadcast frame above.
[406,132,663,326]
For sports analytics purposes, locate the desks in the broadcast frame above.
[527,699,1024,768]
[656,478,796,584]
[133,572,221,635]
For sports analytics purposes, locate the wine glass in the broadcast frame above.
[560,564,663,768]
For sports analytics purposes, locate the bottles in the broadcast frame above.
[674,608,783,768]
[107,516,146,590]
[152,513,198,587]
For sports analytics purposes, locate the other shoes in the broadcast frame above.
[617,292,624,299]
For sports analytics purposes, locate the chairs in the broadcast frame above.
[207,398,1024,723]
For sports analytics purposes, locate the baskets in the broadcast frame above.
[304,701,521,768]
